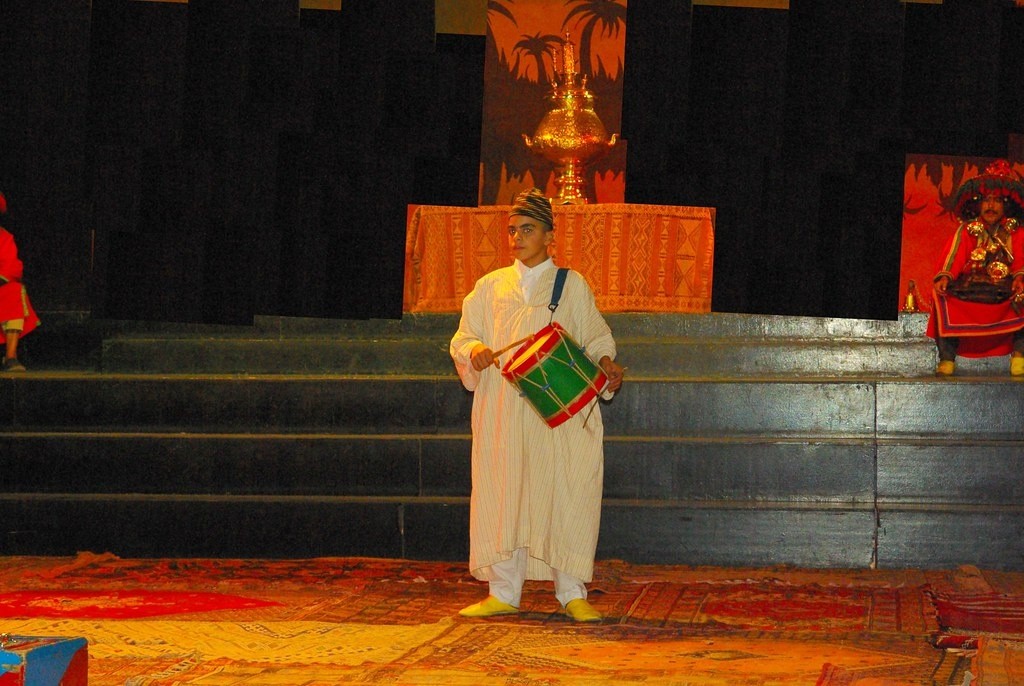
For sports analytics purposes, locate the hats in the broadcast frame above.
[952,159,1024,222]
[508,187,554,225]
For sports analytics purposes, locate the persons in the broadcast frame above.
[923,165,1024,376]
[0,196,42,373]
[448,187,622,620]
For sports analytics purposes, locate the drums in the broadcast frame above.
[501,322,610,429]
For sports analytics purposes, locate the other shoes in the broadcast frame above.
[459,596,518,617]
[1009,357,1023,376]
[566,597,601,622]
[2,357,25,372]
[937,360,956,377]
